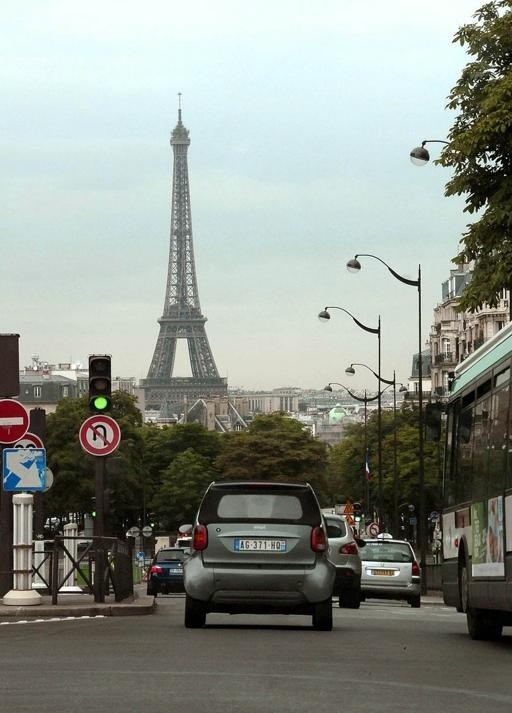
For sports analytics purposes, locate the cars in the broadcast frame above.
[42,511,83,536]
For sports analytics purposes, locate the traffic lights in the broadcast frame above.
[90,356,109,409]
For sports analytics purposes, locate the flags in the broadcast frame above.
[365,451,369,480]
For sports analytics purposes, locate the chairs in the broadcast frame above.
[218,497,302,519]
[362,548,403,561]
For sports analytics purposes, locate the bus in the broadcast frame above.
[441,319,510,638]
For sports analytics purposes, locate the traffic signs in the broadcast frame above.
[11,433,42,448]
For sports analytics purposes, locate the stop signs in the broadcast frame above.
[0,399,29,443]
